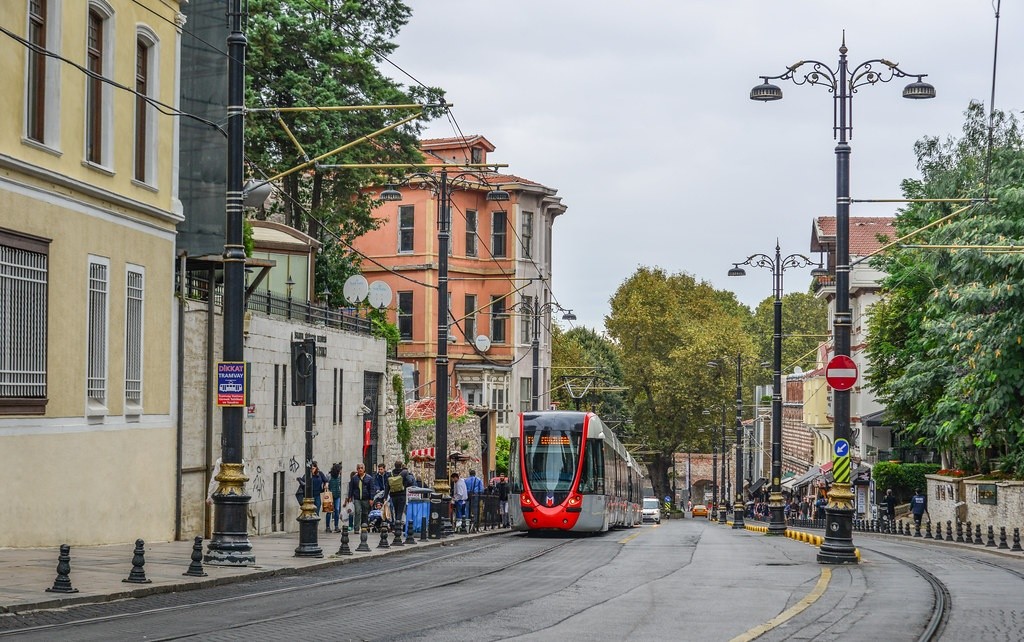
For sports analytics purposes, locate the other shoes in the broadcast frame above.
[326,527,332,533]
[335,528,341,533]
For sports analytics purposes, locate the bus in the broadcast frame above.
[507,374,647,535]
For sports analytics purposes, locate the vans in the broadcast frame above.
[641,497,662,524]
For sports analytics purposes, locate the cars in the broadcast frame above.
[692,505,709,519]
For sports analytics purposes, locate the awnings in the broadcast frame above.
[781,477,800,493]
[748,478,766,494]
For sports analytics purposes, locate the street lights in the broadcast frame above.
[727,239,832,532]
[698,395,747,526]
[493,288,577,411]
[695,420,734,522]
[705,342,773,530]
[749,27,936,566]
[375,166,514,538]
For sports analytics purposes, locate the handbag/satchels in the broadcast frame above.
[465,492,475,501]
[346,501,355,516]
[320,489,335,513]
[380,501,392,522]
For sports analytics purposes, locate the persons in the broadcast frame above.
[816,495,826,519]
[784,501,790,519]
[301,461,326,516]
[680,498,685,511]
[343,463,392,534]
[725,501,731,515]
[800,497,810,519]
[484,473,519,529]
[450,470,484,528]
[390,460,429,529]
[687,499,691,512]
[325,464,342,533]
[881,489,897,519]
[908,488,928,529]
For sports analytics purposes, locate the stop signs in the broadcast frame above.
[824,355,859,391]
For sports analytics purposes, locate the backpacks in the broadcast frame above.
[388,470,404,492]
